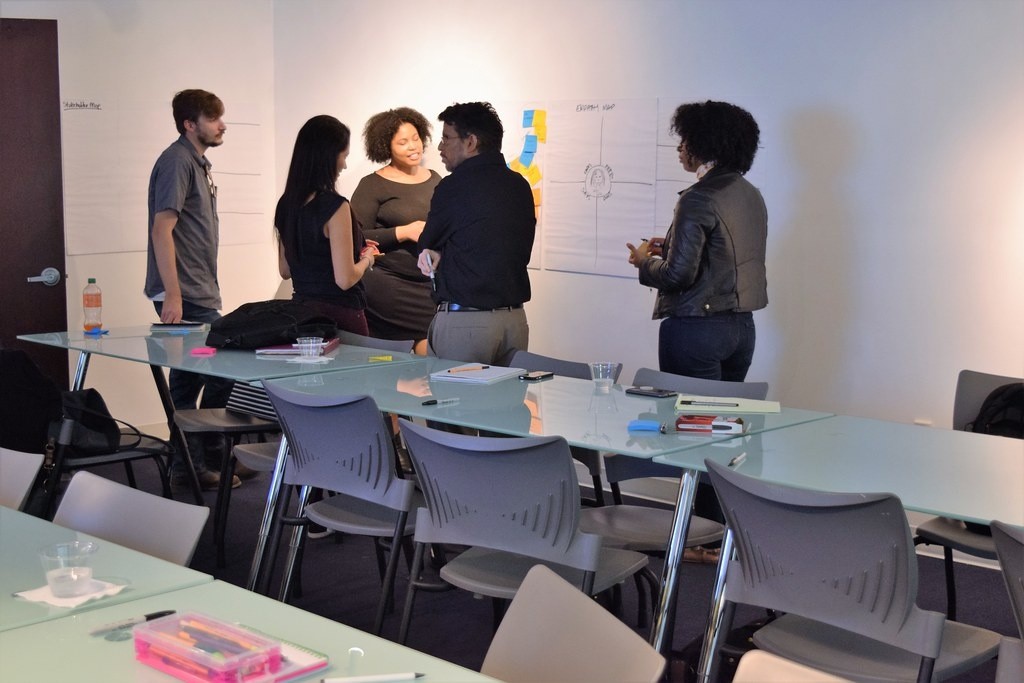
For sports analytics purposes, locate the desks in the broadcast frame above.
[0,577,510,683]
[250,357,839,682]
[16,321,428,593]
[651,418,1024,683]
[0,506,214,633]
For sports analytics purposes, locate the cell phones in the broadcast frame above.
[292,340,329,347]
[626,388,677,397]
[518,371,554,381]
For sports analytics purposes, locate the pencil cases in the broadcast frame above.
[131,611,282,683]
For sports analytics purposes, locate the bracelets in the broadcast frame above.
[363,257,373,272]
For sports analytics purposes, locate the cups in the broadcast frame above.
[297,337,323,359]
[39,541,100,597]
[588,361,618,388]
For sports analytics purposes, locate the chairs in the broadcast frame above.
[0,333,1024,683]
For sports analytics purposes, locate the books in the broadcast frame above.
[674,393,780,413]
[151,323,205,333]
[257,339,342,356]
[431,363,527,385]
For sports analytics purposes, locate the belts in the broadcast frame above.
[440,302,523,312]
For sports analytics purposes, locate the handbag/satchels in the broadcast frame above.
[204,300,336,349]
[62,388,176,456]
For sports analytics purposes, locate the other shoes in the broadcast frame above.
[684,546,720,564]
[307,528,334,538]
[206,456,259,479]
[169,463,242,490]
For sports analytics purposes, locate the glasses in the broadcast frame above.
[441,135,470,144]
[207,167,216,198]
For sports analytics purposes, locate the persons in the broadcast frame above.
[349,108,441,434]
[145,90,241,492]
[416,102,536,579]
[272,115,374,537]
[627,100,769,562]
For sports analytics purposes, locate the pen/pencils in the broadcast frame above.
[727,452,745,466]
[680,400,740,407]
[146,619,268,678]
[659,420,669,434]
[448,366,490,372]
[425,253,436,291]
[320,672,426,683]
[87,610,177,635]
[744,422,752,434]
[421,397,459,406]
[640,238,663,248]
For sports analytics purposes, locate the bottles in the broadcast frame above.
[84,278,103,331]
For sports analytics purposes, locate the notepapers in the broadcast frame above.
[191,347,217,355]
